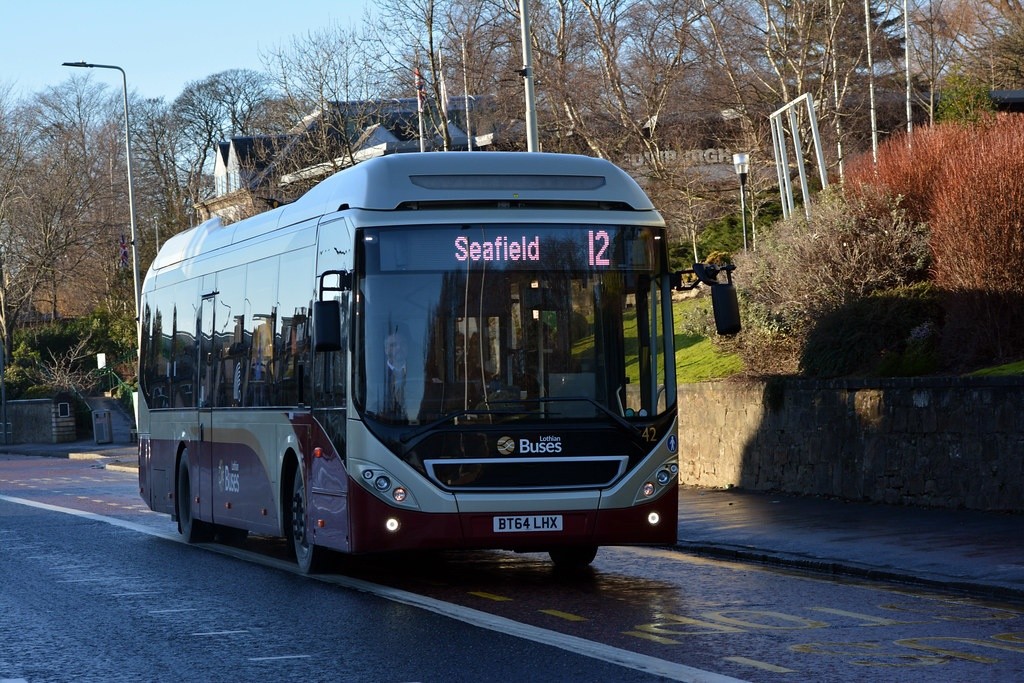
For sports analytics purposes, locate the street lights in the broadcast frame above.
[64,62,142,335]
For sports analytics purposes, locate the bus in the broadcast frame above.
[137,152,741,572]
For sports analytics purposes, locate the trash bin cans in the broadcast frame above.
[91,409,112,444]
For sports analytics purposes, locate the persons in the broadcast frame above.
[371,333,443,409]
[526,319,565,391]
[455,333,481,380]
